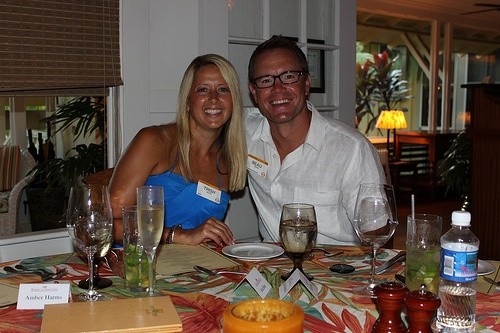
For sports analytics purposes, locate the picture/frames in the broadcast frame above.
[272,33,326,95]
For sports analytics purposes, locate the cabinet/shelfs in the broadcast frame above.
[460,82,500,261]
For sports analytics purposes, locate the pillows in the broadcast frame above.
[0,145,21,191]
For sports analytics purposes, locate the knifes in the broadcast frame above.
[370,251,408,274]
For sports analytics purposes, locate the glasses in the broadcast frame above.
[252,70,307,89]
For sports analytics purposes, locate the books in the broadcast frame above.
[40,296,184,333]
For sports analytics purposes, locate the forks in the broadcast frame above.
[314,246,344,257]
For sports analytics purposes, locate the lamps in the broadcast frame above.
[373,109,408,163]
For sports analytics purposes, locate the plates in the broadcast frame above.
[477,258,496,275]
[221,242,285,262]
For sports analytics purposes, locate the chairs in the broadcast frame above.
[0,147,36,236]
[81,166,115,212]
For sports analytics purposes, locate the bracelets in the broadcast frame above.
[166,224,179,244]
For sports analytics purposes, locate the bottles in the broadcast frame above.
[434,211,479,333]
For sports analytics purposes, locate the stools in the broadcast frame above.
[387,161,417,208]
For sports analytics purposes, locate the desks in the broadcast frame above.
[0,242,500,333]
[393,131,465,203]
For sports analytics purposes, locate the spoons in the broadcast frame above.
[3,265,66,281]
[194,265,247,276]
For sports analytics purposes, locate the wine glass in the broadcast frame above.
[66,185,113,303]
[354,183,396,297]
[280,202,318,283]
[137,185,165,297]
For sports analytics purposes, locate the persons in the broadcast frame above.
[242,34,393,248]
[102,54,247,246]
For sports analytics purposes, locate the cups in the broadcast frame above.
[122,205,156,292]
[405,214,442,297]
